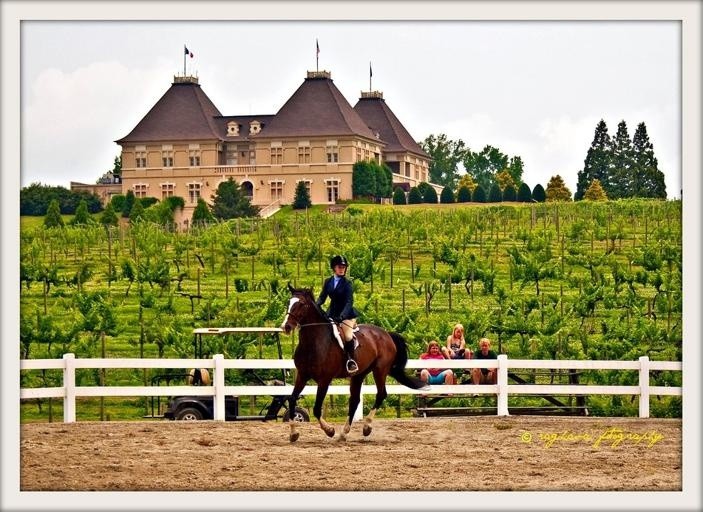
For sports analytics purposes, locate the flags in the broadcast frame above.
[185,48,193,59]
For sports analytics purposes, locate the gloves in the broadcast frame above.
[335,312,343,322]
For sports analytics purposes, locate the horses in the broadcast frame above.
[280,280,431,444]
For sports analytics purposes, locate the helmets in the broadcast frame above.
[330,255,348,269]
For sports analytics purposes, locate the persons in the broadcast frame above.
[419,340,453,397]
[316,255,358,372]
[441,324,471,373]
[472,338,499,397]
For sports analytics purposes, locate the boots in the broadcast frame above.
[345,339,357,377]
